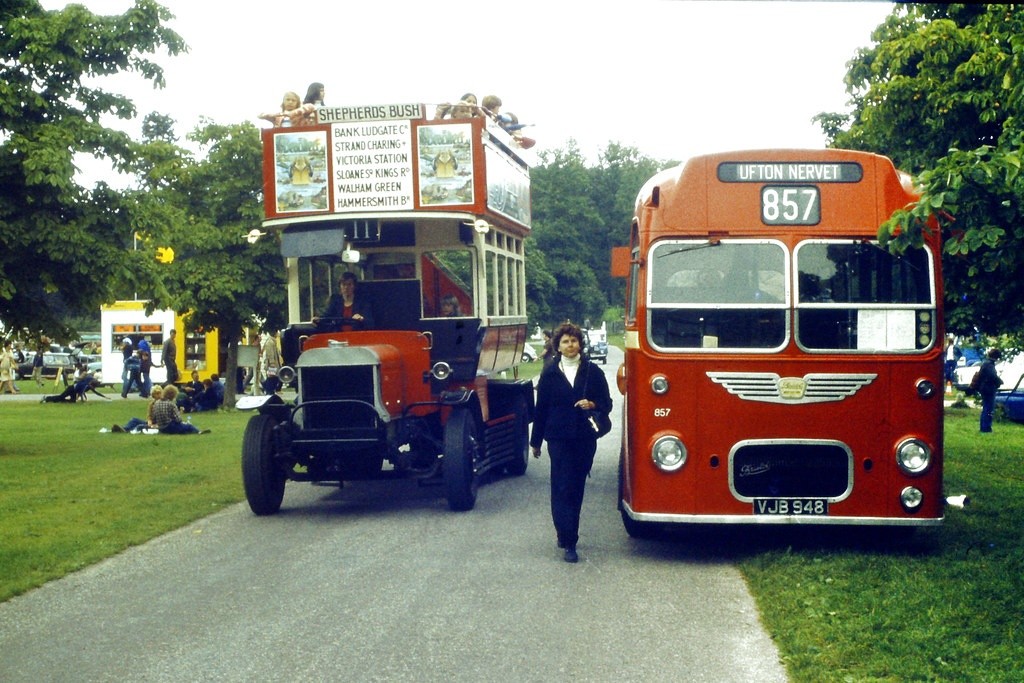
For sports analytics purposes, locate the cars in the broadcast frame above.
[950,349,1024,393]
[987,389,1024,423]
[583,330,608,365]
[518,341,538,366]
[13,351,80,376]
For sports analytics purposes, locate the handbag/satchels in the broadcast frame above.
[588,408,611,438]
[968,372,983,389]
[267,366,278,377]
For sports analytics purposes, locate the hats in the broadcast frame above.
[123,337,133,345]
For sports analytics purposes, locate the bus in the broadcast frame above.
[237,103,544,516]
[613,145,947,558]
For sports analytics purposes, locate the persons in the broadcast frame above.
[975,349,1004,433]
[111,385,164,435]
[311,271,376,332]
[440,294,465,318]
[259,79,537,150]
[0,328,284,413]
[943,337,963,393]
[529,323,614,565]
[152,384,212,436]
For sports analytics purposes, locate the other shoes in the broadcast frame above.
[200,430,212,434]
[112,424,126,434]
[557,539,565,549]
[565,546,578,562]
